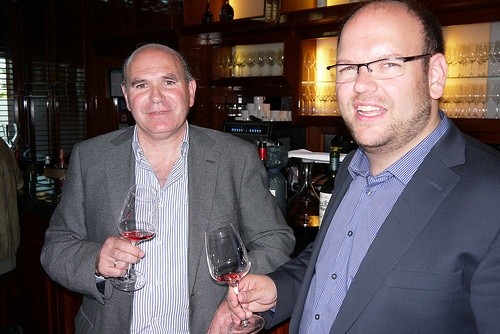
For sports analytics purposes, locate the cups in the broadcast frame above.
[242,96,292,122]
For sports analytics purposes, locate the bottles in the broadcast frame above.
[219,0,234,22]
[202,0,213,23]
[284,158,320,232]
[319,150,341,230]
[58,148,65,169]
[258,141,267,166]
[45,154,51,168]
[268,168,285,219]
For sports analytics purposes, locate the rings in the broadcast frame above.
[114,260,119,267]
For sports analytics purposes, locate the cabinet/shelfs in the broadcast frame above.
[178,0,500,226]
[0,211,82,334]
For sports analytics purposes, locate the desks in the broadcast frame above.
[44,168,67,179]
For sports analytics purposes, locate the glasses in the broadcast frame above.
[326,53,433,83]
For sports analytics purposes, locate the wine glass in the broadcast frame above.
[109,184,160,292]
[301,85,340,116]
[444,39,500,78]
[216,48,284,77]
[205,221,265,334]
[440,83,500,119]
[304,48,337,82]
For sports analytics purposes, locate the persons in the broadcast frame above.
[41,43,297,334]
[225,0,500,334]
[1,138,21,275]
